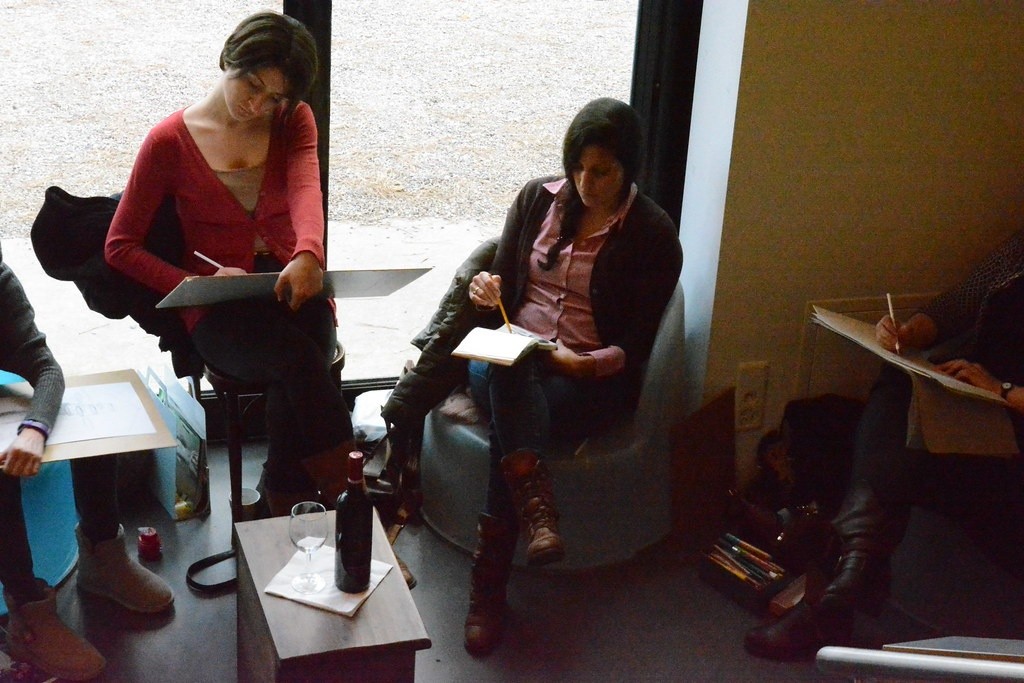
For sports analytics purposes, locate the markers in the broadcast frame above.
[723,533,772,561]
[732,546,767,569]
[708,535,785,591]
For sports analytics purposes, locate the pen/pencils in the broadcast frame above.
[887,293,899,355]
[194,251,224,268]
[489,274,511,333]
[714,545,751,576]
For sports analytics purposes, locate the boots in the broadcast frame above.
[745,544,851,657]
[2,576,106,680]
[821,476,907,607]
[501,446,565,566]
[301,436,368,501]
[264,481,313,516]
[76,522,175,612]
[464,512,518,653]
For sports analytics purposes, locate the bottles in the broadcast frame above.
[333,450,373,593]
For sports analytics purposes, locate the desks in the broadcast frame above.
[233,506,431,683]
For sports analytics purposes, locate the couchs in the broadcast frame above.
[419,238,688,569]
[0,460,79,616]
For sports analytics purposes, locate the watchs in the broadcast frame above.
[1001,381,1012,400]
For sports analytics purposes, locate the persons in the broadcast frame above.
[105,13,418,591]
[464,97,683,653]
[745,230,1024,661]
[0,260,174,680]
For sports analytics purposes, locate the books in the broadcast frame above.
[451,323,557,366]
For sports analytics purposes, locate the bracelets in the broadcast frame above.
[16,420,50,442]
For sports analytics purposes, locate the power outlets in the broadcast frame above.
[735,361,766,431]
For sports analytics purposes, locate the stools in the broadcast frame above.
[203,341,345,548]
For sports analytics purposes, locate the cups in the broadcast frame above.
[228,487,260,521]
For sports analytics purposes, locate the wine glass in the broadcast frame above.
[287,501,327,593]
